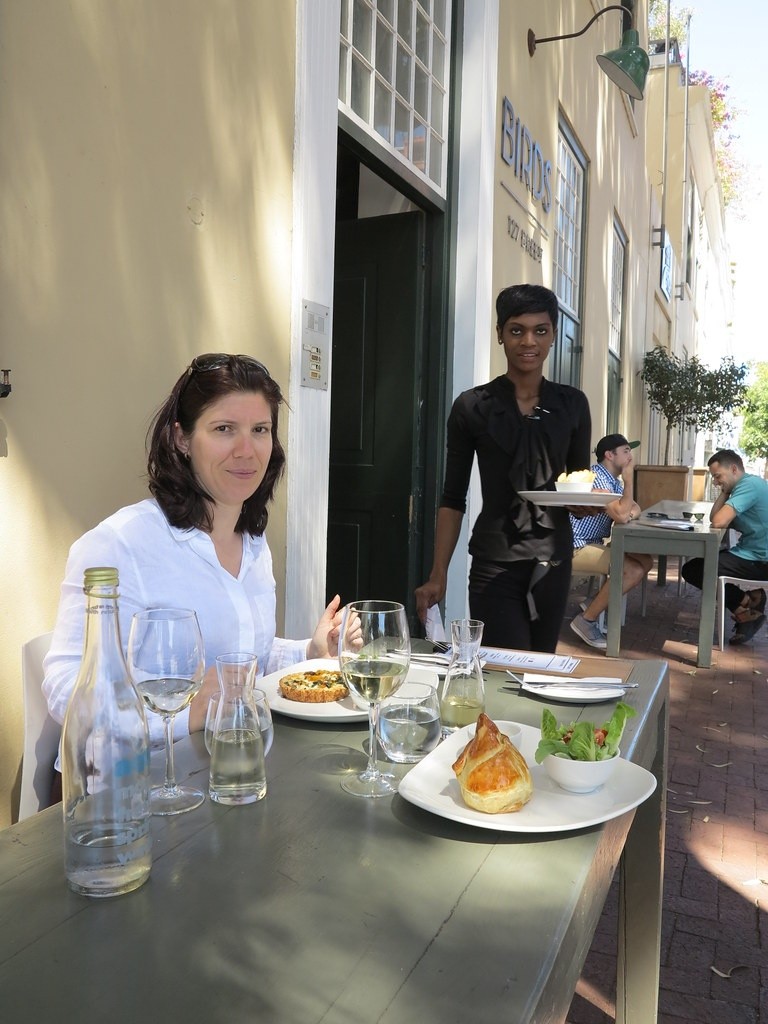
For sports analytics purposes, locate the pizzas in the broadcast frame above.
[279,670,350,703]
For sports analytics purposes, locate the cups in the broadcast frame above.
[204,688,275,761]
[466,723,522,752]
[694,513,705,520]
[683,512,693,518]
[375,682,441,763]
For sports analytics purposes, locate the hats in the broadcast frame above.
[596,434,640,456]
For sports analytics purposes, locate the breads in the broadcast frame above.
[452,713,533,814]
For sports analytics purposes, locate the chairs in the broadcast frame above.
[17,632,63,825]
[569,569,628,631]
[717,575,768,652]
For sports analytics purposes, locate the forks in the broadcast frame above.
[387,649,487,665]
[506,669,638,688]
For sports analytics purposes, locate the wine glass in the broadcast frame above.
[128,607,207,818]
[338,599,411,798]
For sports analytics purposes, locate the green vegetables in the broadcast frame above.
[534,702,638,766]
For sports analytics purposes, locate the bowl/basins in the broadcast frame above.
[538,746,621,792]
[554,482,594,492]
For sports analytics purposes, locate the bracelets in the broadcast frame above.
[630,513,633,518]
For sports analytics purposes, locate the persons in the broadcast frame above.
[569,433,654,649]
[681,449,768,644]
[42,351,363,807]
[413,284,610,652]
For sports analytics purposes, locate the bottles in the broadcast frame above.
[438,620,486,728]
[61,568,153,897]
[208,653,267,805]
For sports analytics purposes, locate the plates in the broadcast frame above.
[253,658,439,722]
[522,678,625,703]
[518,490,624,507]
[398,721,658,833]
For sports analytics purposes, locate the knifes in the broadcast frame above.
[505,681,640,688]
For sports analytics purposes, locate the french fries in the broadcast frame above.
[556,468,596,483]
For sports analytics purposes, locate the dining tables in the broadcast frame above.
[0,636,670,1024]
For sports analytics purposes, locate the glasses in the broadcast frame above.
[683,512,705,520]
[178,353,269,405]
[647,512,668,519]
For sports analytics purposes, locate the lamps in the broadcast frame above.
[528,5,650,101]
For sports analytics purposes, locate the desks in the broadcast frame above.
[606,500,731,669]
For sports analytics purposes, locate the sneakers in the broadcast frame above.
[744,588,767,612]
[729,609,765,644]
[579,598,607,633]
[571,614,608,648]
